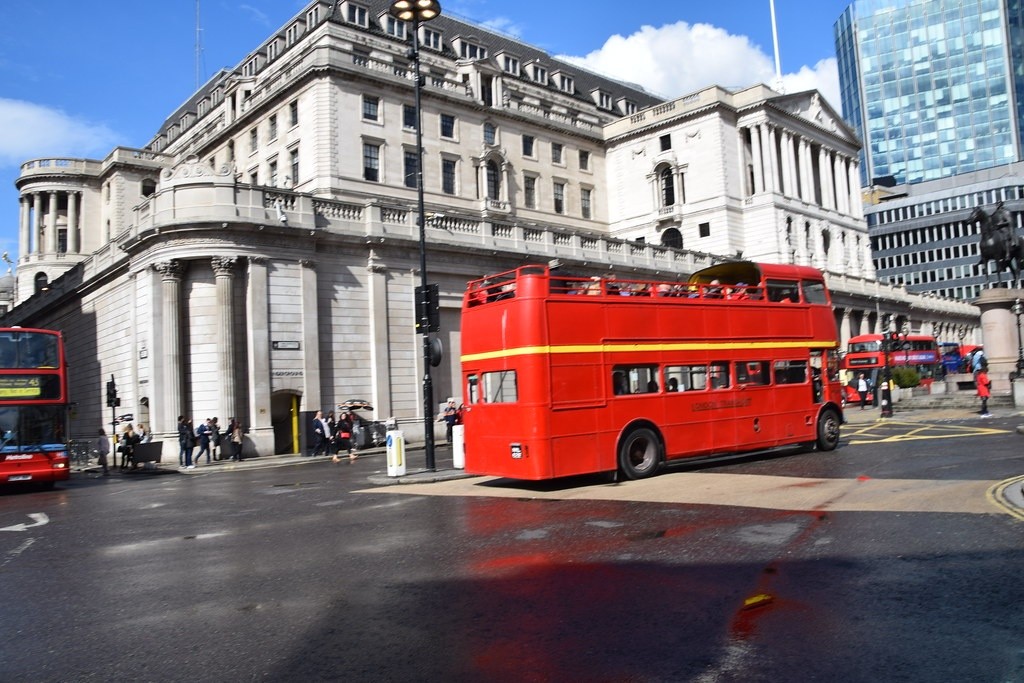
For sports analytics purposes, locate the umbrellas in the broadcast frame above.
[339,399,374,411]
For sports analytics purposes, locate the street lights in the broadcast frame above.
[390,0,444,472]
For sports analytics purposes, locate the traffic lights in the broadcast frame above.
[890,332,901,351]
[107,382,116,407]
[115,398,121,407]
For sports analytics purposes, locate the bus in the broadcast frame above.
[958,345,983,373]
[457,261,847,482]
[842,333,944,404]
[0,325,72,486]
[936,342,961,378]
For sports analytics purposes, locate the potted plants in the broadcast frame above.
[888,365,928,400]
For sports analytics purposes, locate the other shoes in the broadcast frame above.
[350,454,358,459]
[332,456,342,462]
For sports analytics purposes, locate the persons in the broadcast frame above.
[854,373,870,410]
[222,417,244,461]
[194,418,212,466]
[312,408,358,462]
[210,417,221,461]
[480,274,493,288]
[97,429,110,473]
[977,367,991,414]
[177,416,196,469]
[444,401,464,442]
[121,423,151,470]
[989,200,1016,262]
[613,372,624,394]
[647,381,658,392]
[569,272,762,300]
[549,259,602,294]
